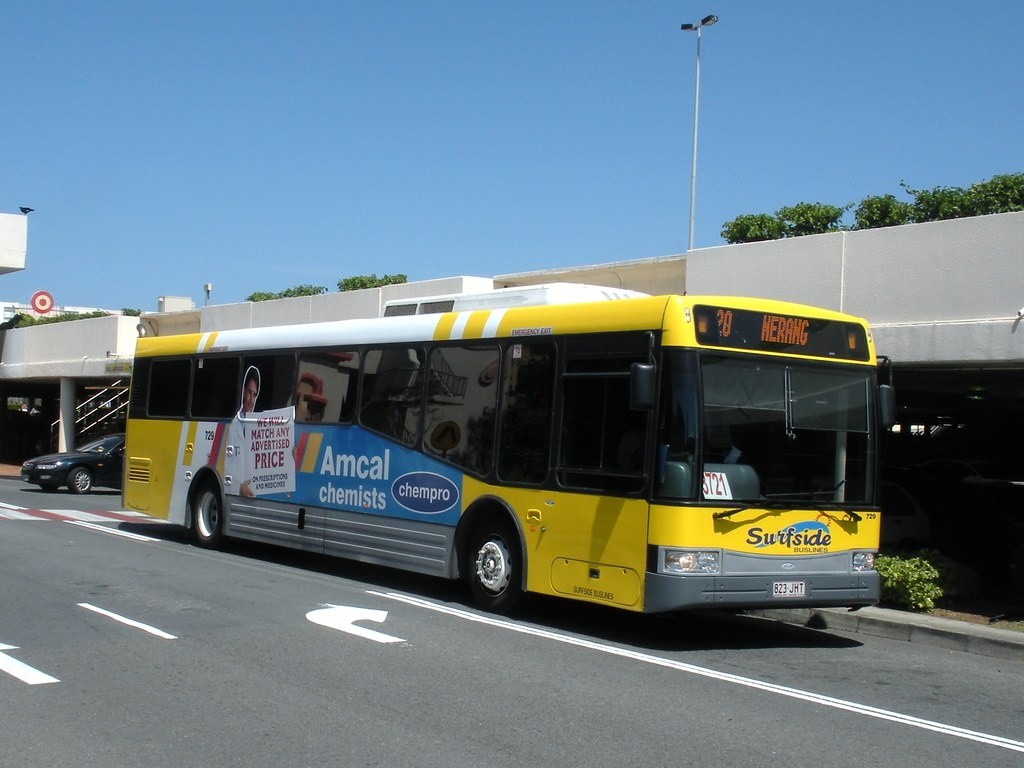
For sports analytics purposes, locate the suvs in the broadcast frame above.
[879,482,931,553]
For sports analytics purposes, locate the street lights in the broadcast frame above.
[681,15,718,250]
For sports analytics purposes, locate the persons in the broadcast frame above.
[19,401,27,412]
[224,368,260,498]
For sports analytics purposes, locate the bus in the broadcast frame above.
[121,282,896,615]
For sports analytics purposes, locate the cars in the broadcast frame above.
[20,433,126,494]
